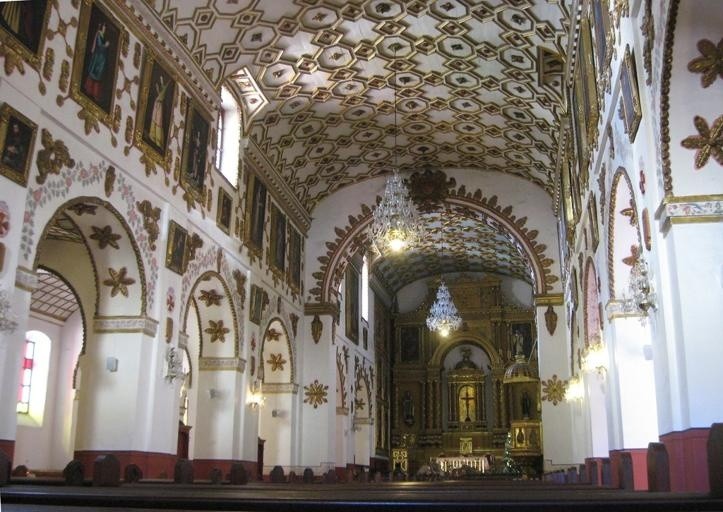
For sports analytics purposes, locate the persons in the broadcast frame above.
[520,391,533,418]
[401,389,416,424]
[510,327,525,356]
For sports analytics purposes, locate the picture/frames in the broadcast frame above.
[556,0,643,324]
[1,103,39,186]
[249,284,264,324]
[63,3,126,129]
[217,174,301,297]
[1,1,55,72]
[165,220,188,276]
[131,45,182,170]
[176,99,214,205]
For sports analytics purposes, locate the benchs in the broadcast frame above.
[0,421,723,511]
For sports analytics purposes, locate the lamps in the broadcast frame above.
[368,46,430,251]
[208,389,215,400]
[272,411,277,416]
[584,349,609,377]
[628,256,660,318]
[502,334,540,384]
[426,212,462,337]
[564,384,584,402]
[107,357,117,372]
